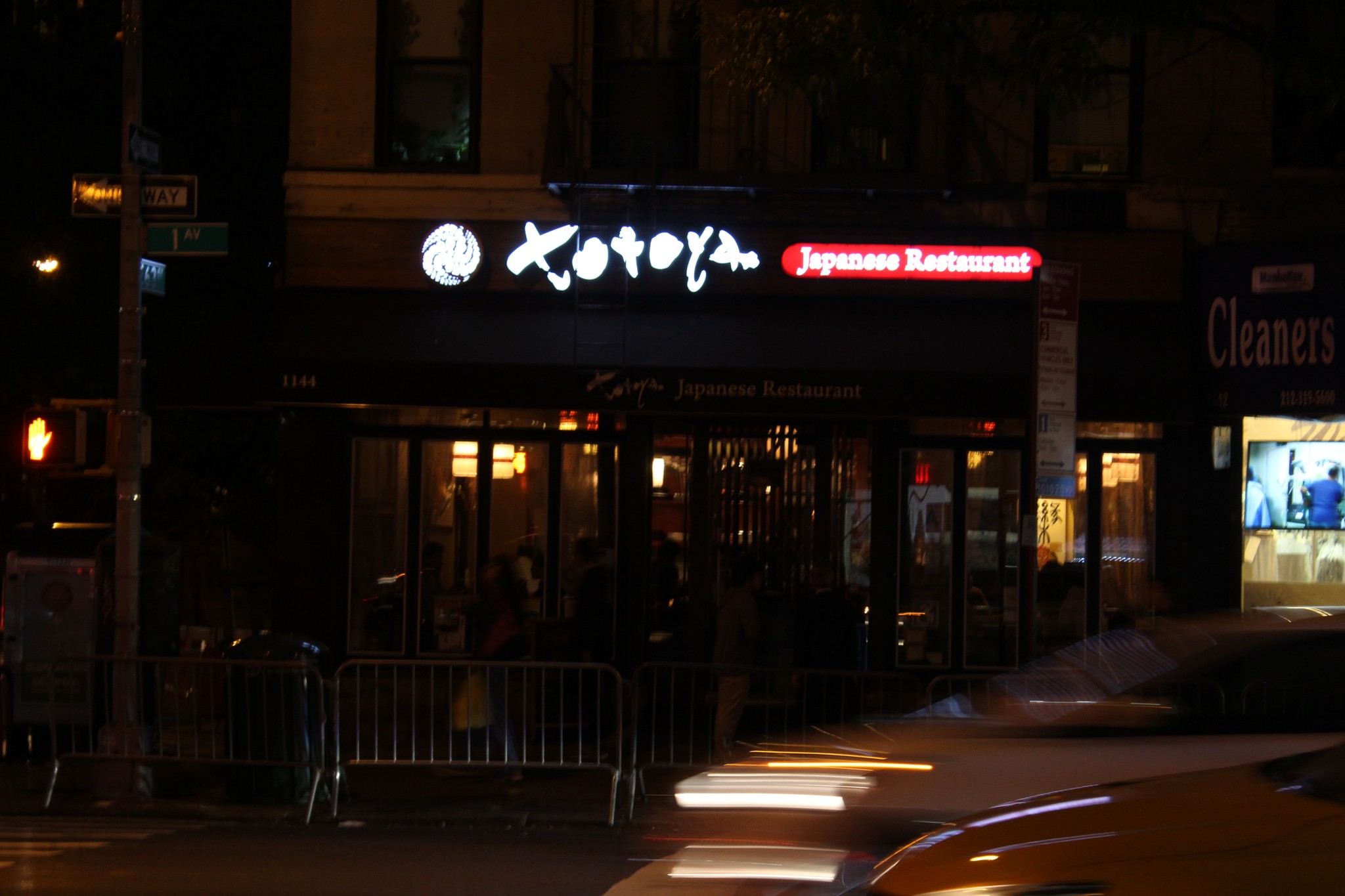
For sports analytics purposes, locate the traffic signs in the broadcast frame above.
[71,172,198,219]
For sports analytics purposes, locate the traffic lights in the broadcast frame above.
[21,407,84,471]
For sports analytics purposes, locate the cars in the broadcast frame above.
[598,600,1344,896]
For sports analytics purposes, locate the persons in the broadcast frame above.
[422,525,764,759]
[1243,467,1344,529]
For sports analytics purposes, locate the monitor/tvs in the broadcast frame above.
[1243,439,1345,531]
